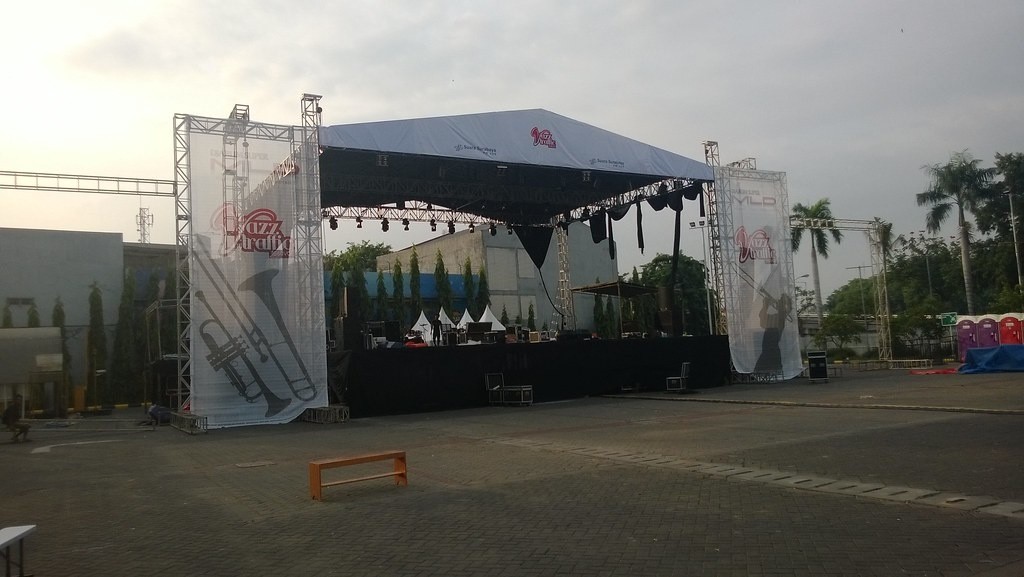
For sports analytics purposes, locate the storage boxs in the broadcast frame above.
[666,362,691,390]
[441,321,549,346]
[485,371,534,405]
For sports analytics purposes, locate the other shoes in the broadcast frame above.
[11,437,19,441]
[22,438,32,442]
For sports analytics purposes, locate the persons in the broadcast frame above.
[0,394,32,443]
[430,314,443,347]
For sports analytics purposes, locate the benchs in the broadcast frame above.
[309,449,407,500]
[0,524,37,577]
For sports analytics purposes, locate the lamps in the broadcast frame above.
[698,220,703,225]
[690,221,694,227]
[329,216,512,236]
